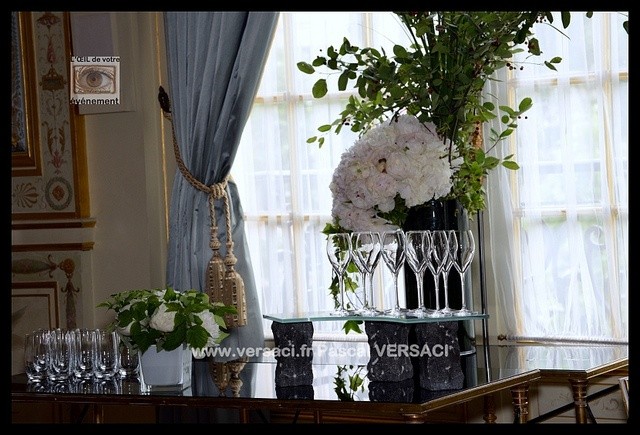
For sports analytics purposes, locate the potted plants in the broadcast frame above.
[137,343,192,387]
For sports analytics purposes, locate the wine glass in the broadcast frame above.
[356,232,380,317]
[326,233,353,317]
[404,231,432,319]
[380,230,405,316]
[349,232,373,317]
[431,230,457,316]
[448,230,478,317]
[422,231,449,319]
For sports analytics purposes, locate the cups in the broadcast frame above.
[24,328,140,383]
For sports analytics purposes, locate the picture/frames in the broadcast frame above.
[10,12,45,177]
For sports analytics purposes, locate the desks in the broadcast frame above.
[12,361,541,424]
[218,340,629,423]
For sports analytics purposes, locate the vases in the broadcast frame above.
[400,197,476,357]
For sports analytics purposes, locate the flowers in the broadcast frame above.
[94,286,240,357]
[296,11,629,335]
